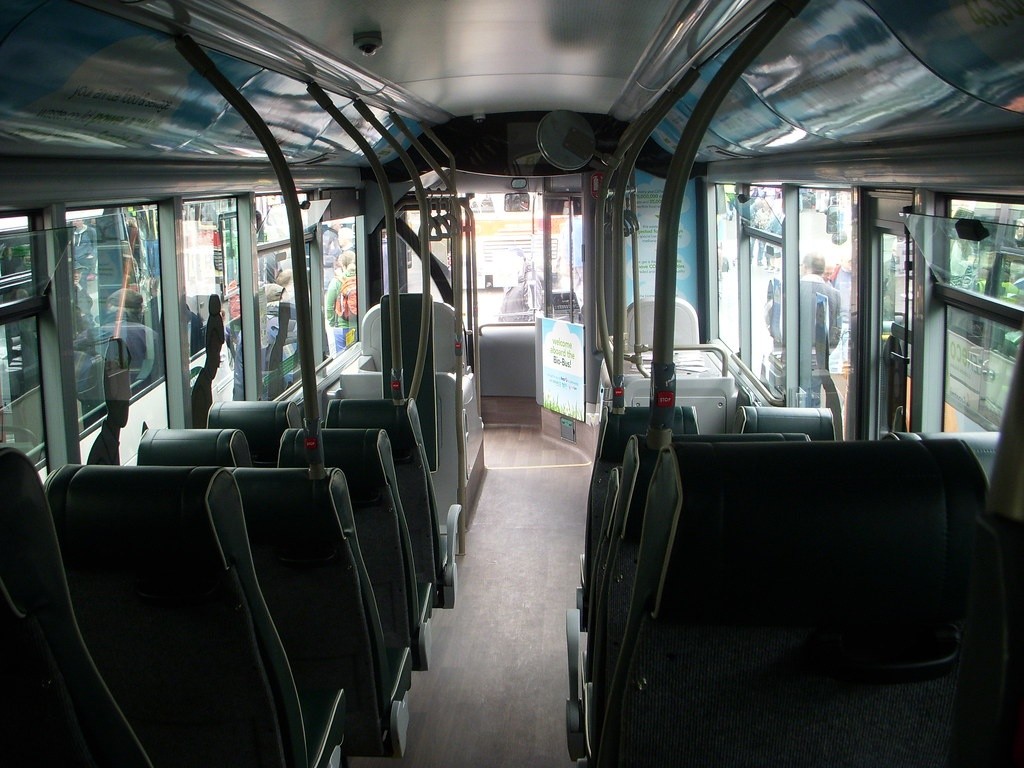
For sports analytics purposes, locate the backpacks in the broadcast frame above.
[263,319,296,390]
[335,272,357,320]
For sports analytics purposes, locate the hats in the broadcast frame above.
[75,261,88,273]
[107,287,143,308]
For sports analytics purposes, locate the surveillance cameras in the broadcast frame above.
[353,38,382,56]
[473,114,487,124]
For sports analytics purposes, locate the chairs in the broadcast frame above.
[0,393,461,768]
[563,404,1024,768]
[625,298,699,348]
[360,296,460,370]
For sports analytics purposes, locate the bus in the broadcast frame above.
[0,191,310,481]
[872,196,1024,432]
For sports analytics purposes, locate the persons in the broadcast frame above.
[499,248,544,322]
[69,219,97,331]
[563,215,583,292]
[126,217,140,266]
[324,220,342,293]
[801,252,842,408]
[326,249,357,354]
[333,227,355,258]
[749,197,782,272]
[0,243,31,303]
[228,280,241,337]
[73,288,159,425]
[233,269,330,402]
